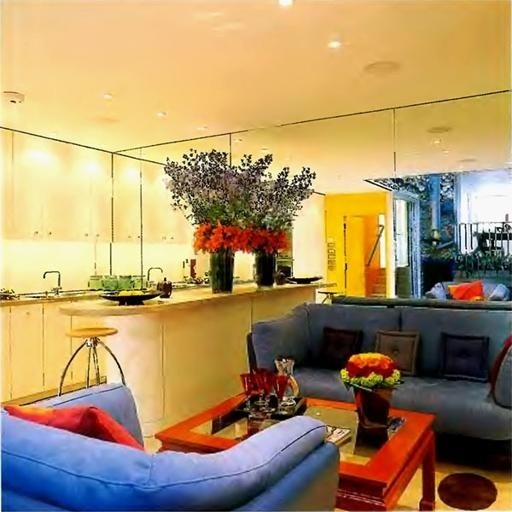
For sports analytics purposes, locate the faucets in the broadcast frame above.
[43,270,62,297]
[146,267,163,281]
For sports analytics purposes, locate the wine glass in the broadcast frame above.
[236,356,302,419]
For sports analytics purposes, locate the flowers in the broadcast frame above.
[163,148,273,288]
[342,352,402,389]
[243,166,316,282]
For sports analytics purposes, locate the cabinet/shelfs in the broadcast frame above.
[0,129,113,242]
[114,155,193,245]
[2,300,145,401]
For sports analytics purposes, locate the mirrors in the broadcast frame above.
[112,89,512,301]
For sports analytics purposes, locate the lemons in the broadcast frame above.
[117,290,144,296]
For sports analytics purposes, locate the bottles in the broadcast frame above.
[156,277,173,298]
[86,274,146,292]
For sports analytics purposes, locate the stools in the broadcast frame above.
[60,327,126,396]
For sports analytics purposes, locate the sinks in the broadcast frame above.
[20,289,97,299]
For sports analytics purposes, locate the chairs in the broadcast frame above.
[426,282,509,301]
[1,383,339,511]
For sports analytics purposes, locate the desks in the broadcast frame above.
[476,232,511,251]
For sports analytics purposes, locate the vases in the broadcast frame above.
[209,250,234,293]
[353,388,392,447]
[255,251,277,286]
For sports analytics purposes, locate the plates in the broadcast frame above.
[287,277,325,284]
[99,294,164,306]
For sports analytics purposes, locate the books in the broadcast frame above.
[319,425,352,447]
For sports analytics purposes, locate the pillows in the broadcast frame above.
[7,402,141,450]
[310,304,511,381]
[449,282,483,300]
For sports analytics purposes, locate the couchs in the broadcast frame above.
[247,302,512,472]
